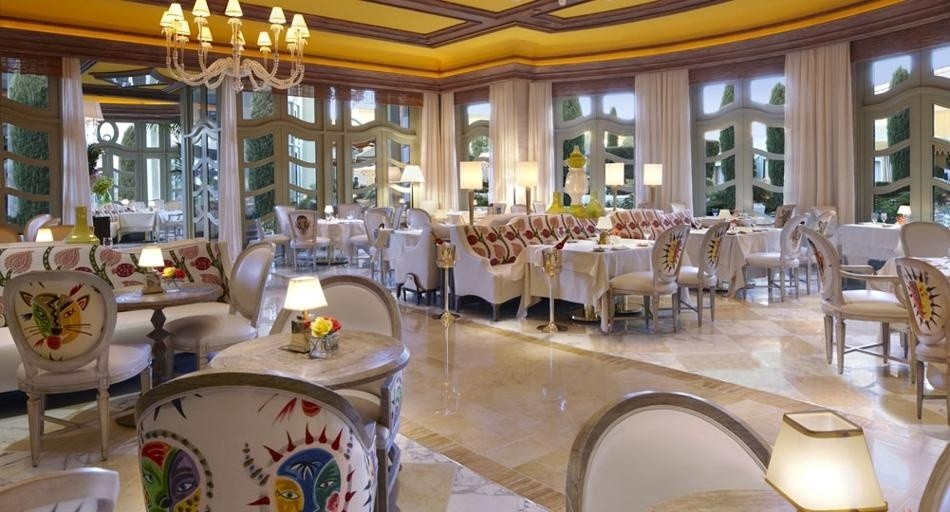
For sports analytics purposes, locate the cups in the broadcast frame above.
[144,270,186,294]
[346,210,356,220]
[103,237,113,249]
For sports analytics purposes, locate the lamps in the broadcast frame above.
[606,161,625,210]
[516,161,542,213]
[401,164,424,205]
[643,162,664,208]
[455,160,482,226]
[157,1,311,94]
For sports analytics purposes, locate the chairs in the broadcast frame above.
[805,204,950,419]
[0,203,407,512]
[566,390,950,511]
[387,206,836,336]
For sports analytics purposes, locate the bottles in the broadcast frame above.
[16,232,24,242]
[286,314,340,358]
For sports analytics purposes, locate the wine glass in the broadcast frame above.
[870,213,879,225]
[712,208,718,218]
[880,212,888,227]
[407,217,415,232]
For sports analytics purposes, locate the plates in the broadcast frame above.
[566,240,579,243]
[593,241,656,253]
[541,242,558,246]
